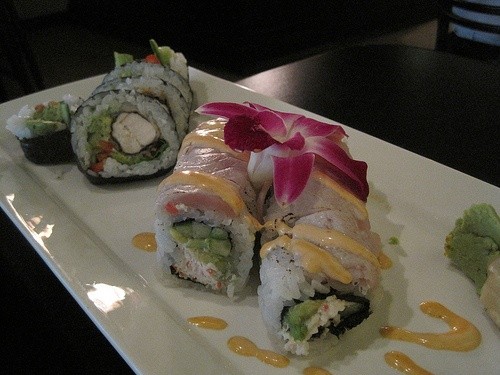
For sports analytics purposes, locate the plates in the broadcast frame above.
[0,58,500,375]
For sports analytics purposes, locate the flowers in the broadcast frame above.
[194,97,371,218]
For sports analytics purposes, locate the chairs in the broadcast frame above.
[435,0,500,59]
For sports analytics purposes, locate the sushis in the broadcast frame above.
[4,38,393,362]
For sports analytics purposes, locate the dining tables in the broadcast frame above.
[0,42,500,375]
